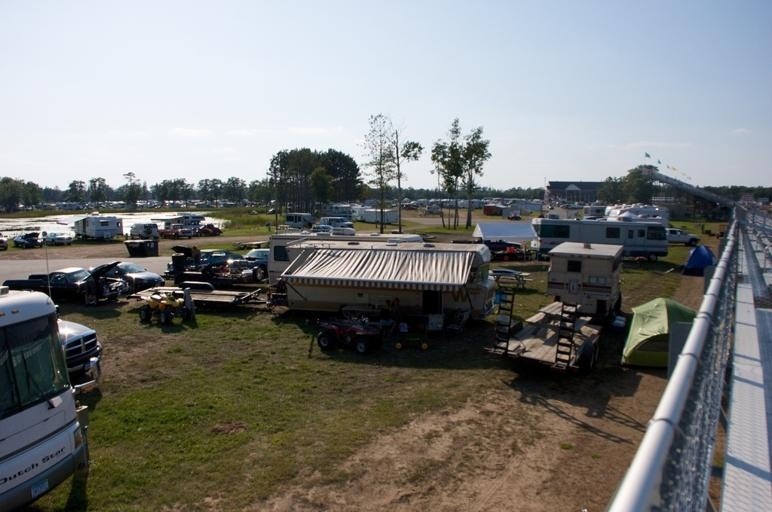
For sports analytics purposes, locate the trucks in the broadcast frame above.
[481,241,624,379]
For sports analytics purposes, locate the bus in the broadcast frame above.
[0,286,101,512]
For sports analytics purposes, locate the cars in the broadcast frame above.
[3,247,269,375]
[17,199,260,211]
[130,224,221,240]
[561,200,610,208]
[401,195,491,215]
[666,227,701,246]
[0,230,73,251]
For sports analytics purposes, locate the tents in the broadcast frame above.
[681,243,718,276]
[620,298,697,367]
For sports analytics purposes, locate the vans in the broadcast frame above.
[321,216,353,228]
